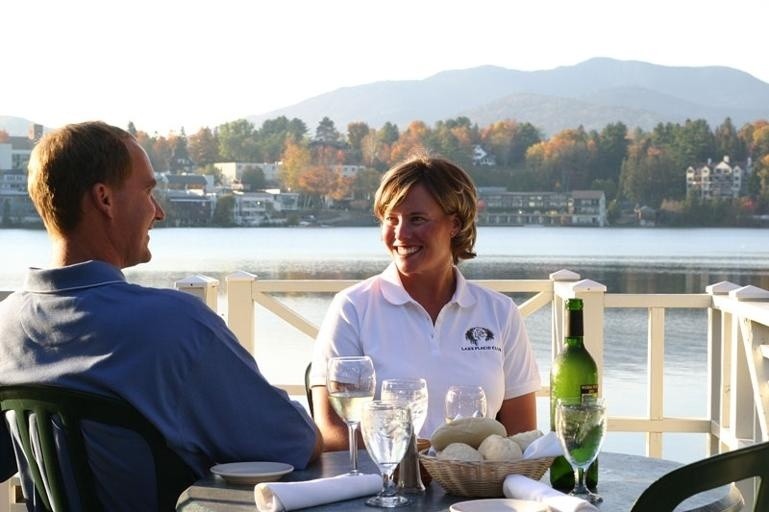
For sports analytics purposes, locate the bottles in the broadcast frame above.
[549,298,601,492]
[393,427,427,494]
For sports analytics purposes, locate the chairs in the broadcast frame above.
[628,439,769,511]
[0,383,225,511]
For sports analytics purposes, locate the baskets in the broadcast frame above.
[416,447,556,499]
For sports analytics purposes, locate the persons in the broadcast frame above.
[309,152,542,451]
[0,121,325,512]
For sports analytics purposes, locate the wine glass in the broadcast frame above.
[326,356,376,477]
[359,403,414,508]
[556,397,607,505]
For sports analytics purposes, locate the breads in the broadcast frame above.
[429,417,545,462]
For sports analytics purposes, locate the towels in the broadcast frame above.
[249,465,385,512]
[497,468,602,512]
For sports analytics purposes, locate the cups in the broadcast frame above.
[445,383,487,425]
[389,439,433,489]
[380,377,430,438]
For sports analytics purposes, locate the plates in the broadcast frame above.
[449,498,549,511]
[210,461,295,486]
